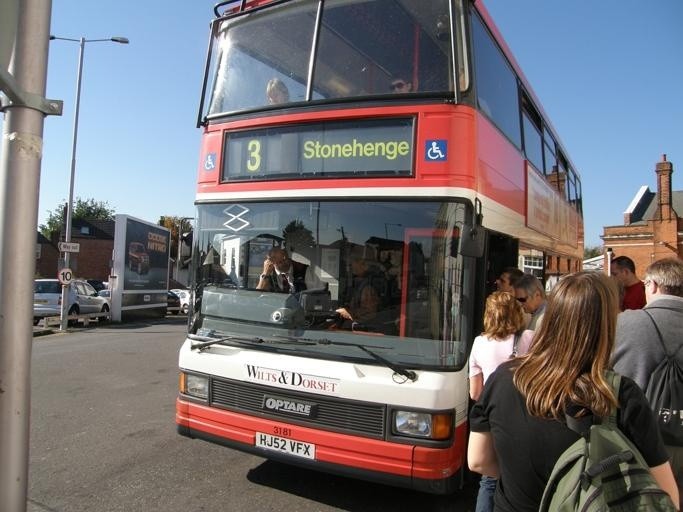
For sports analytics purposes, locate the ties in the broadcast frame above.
[282,274,290,293]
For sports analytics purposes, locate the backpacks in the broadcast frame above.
[537,370,679,512]
[643,307,683,445]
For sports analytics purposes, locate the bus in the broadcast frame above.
[177,0,586,494]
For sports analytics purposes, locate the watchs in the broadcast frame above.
[261,275,271,279]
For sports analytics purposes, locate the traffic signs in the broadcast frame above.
[57,268,74,284]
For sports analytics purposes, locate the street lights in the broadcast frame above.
[49,36,129,330]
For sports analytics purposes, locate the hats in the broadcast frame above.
[341,245,382,266]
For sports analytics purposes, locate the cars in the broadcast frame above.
[129,242,150,274]
[167,290,192,314]
[34,279,110,325]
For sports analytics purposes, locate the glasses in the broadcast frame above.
[389,81,409,91]
[516,294,529,303]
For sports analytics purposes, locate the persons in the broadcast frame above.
[468,253,682,512]
[384,70,415,94]
[255,246,322,292]
[258,77,290,106]
[335,244,400,335]
